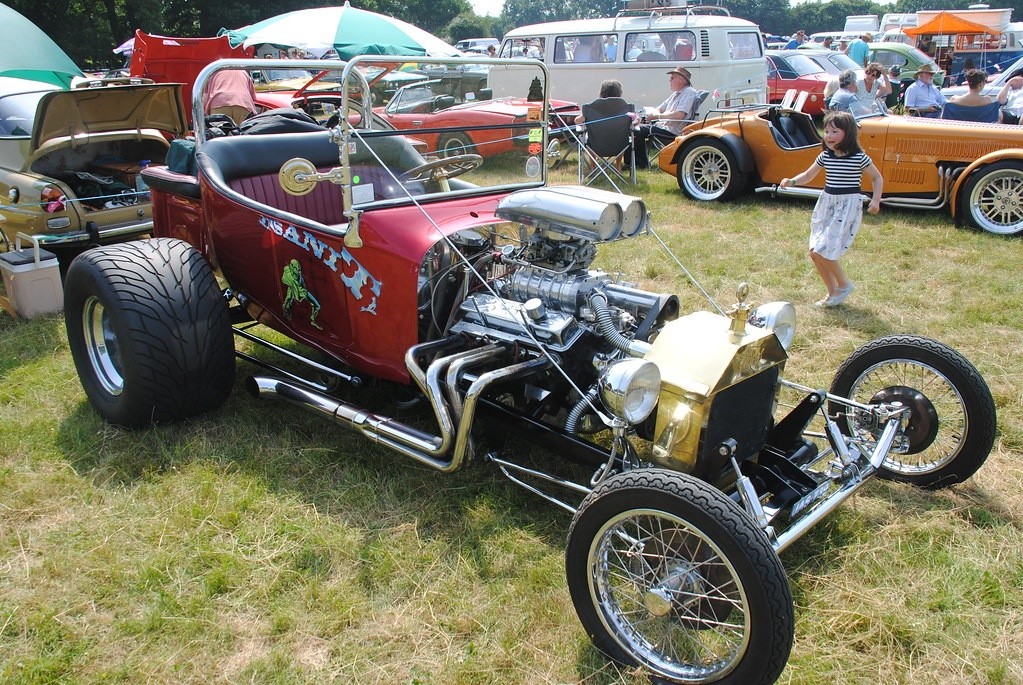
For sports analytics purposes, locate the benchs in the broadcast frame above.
[196,127,435,234]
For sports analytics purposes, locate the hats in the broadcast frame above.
[889,65,902,74]
[913,64,936,79]
[667,66,693,86]
[864,33,872,41]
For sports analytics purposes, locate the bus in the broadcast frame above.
[479,3,770,125]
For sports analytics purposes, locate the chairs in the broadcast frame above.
[764,109,811,150]
[646,90,709,173]
[578,111,638,187]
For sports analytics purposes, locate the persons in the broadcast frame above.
[835,36,851,51]
[623,67,698,171]
[488,45,497,58]
[822,35,834,48]
[573,36,599,63]
[829,69,872,115]
[996,69,1023,126]
[266,51,304,59]
[882,64,902,102]
[604,36,617,62]
[856,62,893,102]
[950,69,1003,121]
[205,70,258,124]
[905,64,949,119]
[575,80,640,171]
[845,32,872,69]
[783,30,804,50]
[780,112,883,309]
[564,38,570,51]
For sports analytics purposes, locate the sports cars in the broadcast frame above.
[648,88,1023,238]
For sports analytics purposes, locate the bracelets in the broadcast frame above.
[790,179,796,185]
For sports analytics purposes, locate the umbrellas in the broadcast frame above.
[0,2,89,89]
[113,34,180,57]
[217,1,465,72]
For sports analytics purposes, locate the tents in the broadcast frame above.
[902,10,1001,71]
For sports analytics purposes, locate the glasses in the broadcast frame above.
[868,72,876,77]
[670,77,681,80]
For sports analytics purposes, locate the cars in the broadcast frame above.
[1,34,678,252]
[65,53,997,685]
[759,5,1023,118]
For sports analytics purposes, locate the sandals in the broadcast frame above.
[816,293,830,308]
[830,282,853,307]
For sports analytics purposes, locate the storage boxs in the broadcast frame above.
[0,231,65,322]
